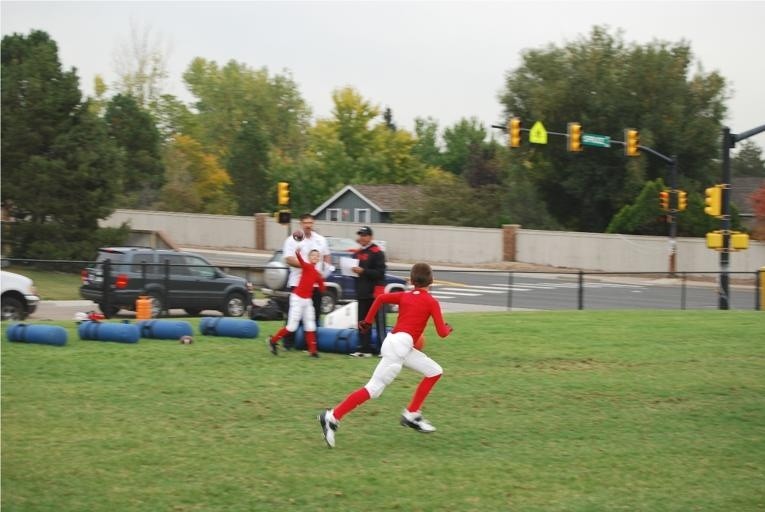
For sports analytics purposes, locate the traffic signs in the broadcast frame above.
[580,132,611,149]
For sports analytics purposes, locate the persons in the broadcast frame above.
[267,245,328,359]
[349,226,387,357]
[317,263,454,448]
[283,213,332,351]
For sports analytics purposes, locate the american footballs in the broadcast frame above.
[294,231,304,241]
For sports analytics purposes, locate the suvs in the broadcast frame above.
[76,245,253,318]
[261,241,409,315]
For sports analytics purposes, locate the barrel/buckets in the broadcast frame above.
[325,302,359,330]
[136,296,151,320]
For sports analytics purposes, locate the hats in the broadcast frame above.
[357,226,372,235]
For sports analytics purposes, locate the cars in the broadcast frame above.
[0,268,39,321]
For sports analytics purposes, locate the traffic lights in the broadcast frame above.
[627,129,640,155]
[276,210,291,225]
[510,118,522,148]
[279,182,291,205]
[678,191,689,211]
[702,186,721,215]
[568,123,584,152]
[658,191,669,212]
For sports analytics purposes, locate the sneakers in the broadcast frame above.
[265,334,279,356]
[311,352,320,358]
[316,407,338,451]
[349,351,374,359]
[399,408,436,435]
